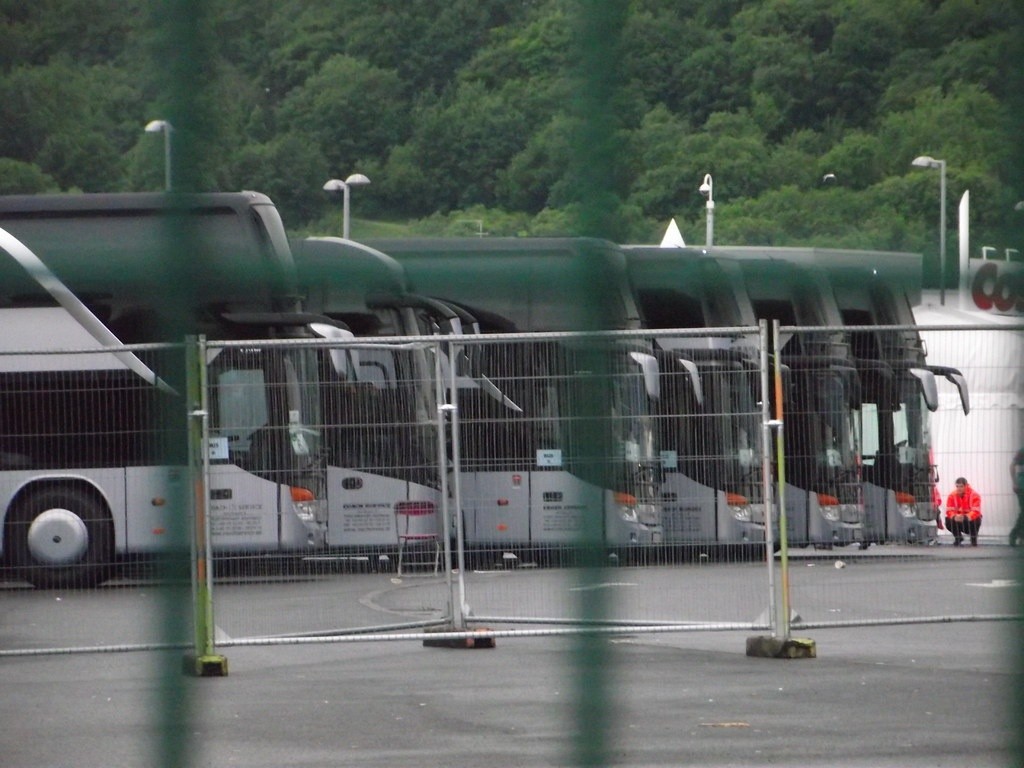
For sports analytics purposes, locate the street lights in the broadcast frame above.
[144,119,172,193]
[910,156,947,307]
[700,173,714,247]
[323,174,370,241]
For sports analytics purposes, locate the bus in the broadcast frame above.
[1,187,975,590]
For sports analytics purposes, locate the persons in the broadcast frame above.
[945,478,982,547]
[934,485,944,546]
[1008,447,1024,546]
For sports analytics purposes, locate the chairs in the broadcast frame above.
[394,501,445,576]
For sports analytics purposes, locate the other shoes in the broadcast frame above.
[953,536,964,546]
[971,537,977,546]
[1008,532,1016,547]
